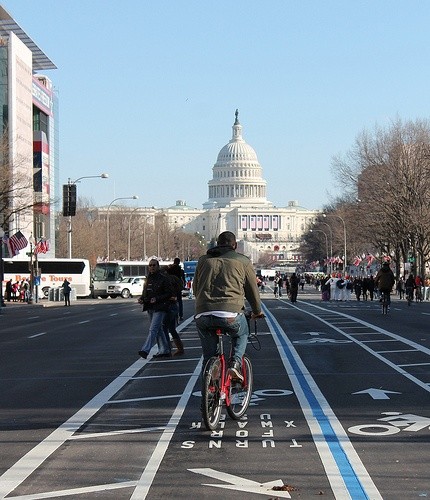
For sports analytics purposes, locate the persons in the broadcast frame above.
[375,262,394,311]
[6,278,31,304]
[256,271,430,303]
[62,279,71,306]
[137,257,187,359]
[192,231,263,414]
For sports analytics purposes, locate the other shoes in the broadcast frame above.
[228,359,244,383]
[154,354,169,360]
[379,297,383,303]
[138,351,148,359]
[387,309,390,311]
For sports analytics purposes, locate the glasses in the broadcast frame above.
[149,264,157,267]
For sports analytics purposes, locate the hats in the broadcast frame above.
[383,262,388,264]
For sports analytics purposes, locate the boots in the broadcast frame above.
[168,342,173,359]
[173,337,184,355]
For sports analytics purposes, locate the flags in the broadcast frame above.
[3,230,28,258]
[33,241,49,255]
[367,253,375,268]
[353,257,360,267]
[311,256,343,267]
[383,256,392,268]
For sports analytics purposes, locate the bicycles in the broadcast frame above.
[376,283,393,315]
[201,310,267,430]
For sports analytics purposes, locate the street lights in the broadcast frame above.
[157,221,204,261]
[143,212,167,260]
[106,195,139,261]
[28,232,36,304]
[311,222,332,274]
[67,173,109,258]
[310,229,328,274]
[126,206,157,260]
[322,214,347,276]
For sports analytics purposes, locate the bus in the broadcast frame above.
[2,258,92,299]
[93,261,198,300]
